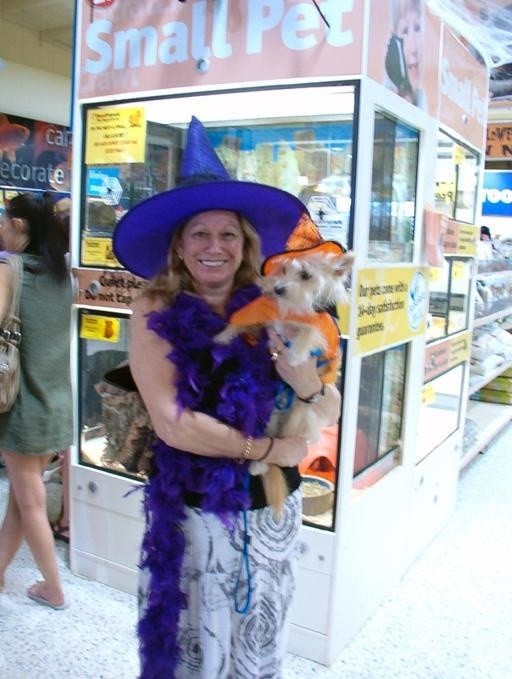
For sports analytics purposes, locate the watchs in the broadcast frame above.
[296,380,326,406]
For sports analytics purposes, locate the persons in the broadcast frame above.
[0,189,78,616]
[38,445,70,547]
[122,211,348,679]
[387,0,430,111]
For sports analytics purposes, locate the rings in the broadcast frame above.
[269,351,280,362]
[274,343,287,353]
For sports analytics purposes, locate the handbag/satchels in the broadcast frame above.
[0,336,21,414]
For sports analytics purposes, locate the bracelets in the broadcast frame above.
[232,433,258,464]
[257,436,276,462]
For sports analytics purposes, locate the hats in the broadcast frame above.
[112,116,313,281]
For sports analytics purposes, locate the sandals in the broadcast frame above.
[50,520,69,543]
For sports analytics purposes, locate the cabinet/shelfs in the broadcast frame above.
[456,255,511,475]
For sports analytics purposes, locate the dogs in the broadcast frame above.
[211,247,358,522]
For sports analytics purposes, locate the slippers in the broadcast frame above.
[28,581,69,610]
